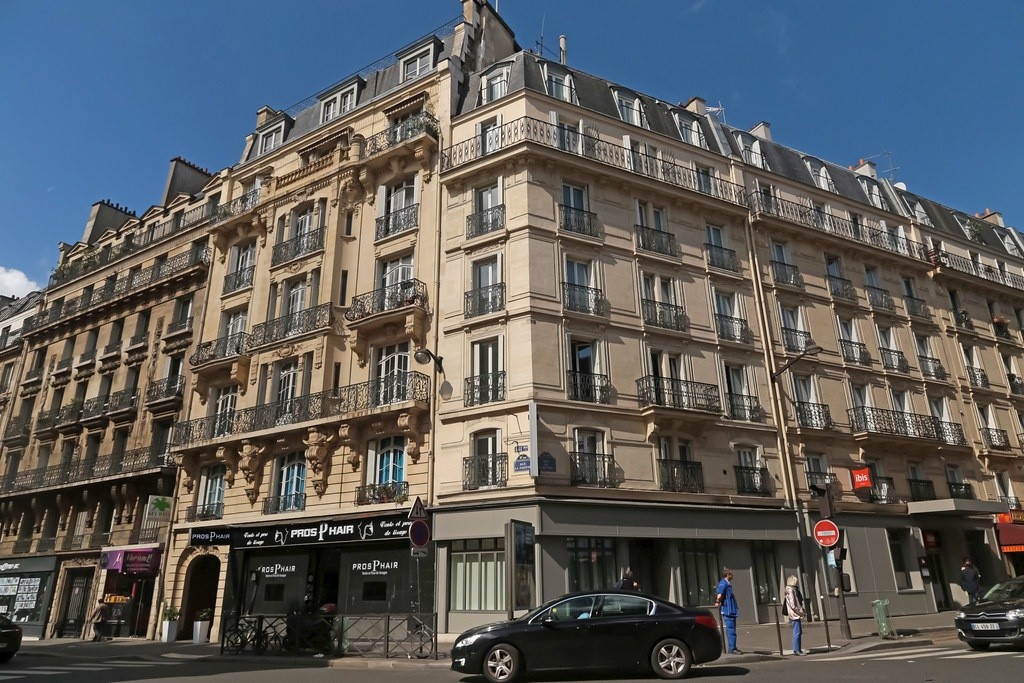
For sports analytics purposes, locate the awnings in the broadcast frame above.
[995,523,1024,545]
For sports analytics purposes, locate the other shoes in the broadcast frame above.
[728,648,744,654]
[92,639,97,642]
[98,635,101,642]
[794,650,807,656]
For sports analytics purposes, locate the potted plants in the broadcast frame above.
[162,608,178,643]
[193,608,211,644]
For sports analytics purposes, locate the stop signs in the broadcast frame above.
[813,520,839,547]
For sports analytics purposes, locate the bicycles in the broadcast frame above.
[410,622,434,660]
[225,616,284,657]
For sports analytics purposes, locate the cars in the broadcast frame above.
[0,613,23,666]
[953,574,1024,650]
[450,587,724,683]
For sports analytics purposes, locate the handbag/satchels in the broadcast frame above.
[781,598,789,616]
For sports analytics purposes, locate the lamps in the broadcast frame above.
[414,348,443,373]
[770,339,823,379]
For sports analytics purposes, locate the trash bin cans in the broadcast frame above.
[870,599,899,640]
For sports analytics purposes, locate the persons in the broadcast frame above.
[785,575,808,656]
[716,569,742,655]
[960,559,982,604]
[89,599,108,642]
[616,573,638,591]
[576,603,590,619]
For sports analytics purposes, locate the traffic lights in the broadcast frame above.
[834,548,848,560]
